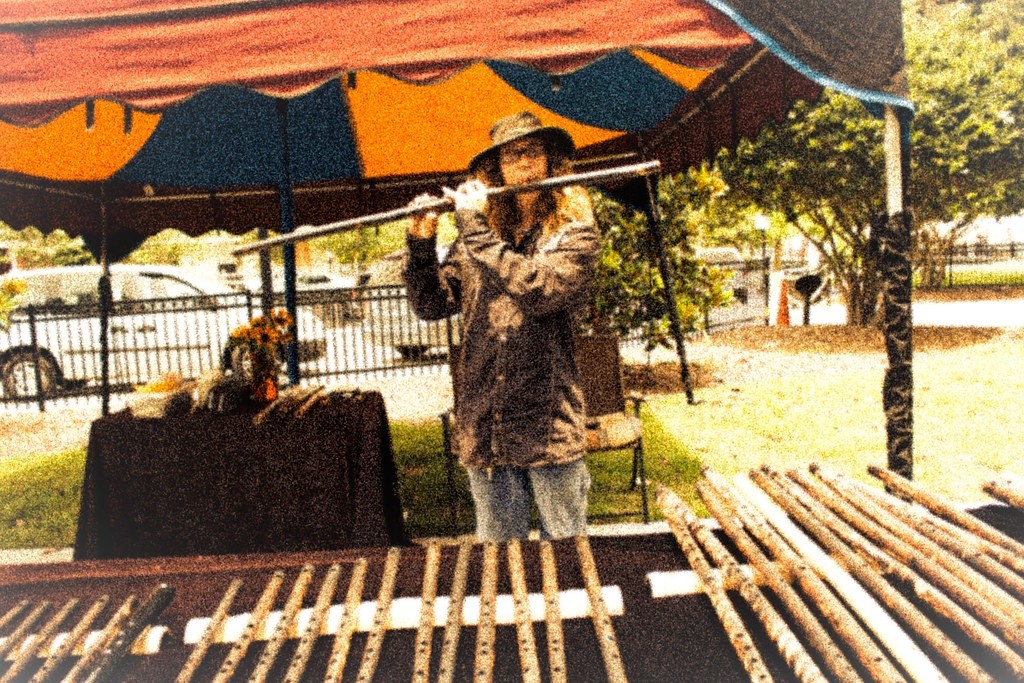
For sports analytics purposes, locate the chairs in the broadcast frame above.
[575,332,650,524]
[437,343,464,520]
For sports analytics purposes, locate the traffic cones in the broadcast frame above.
[772,282,790,325]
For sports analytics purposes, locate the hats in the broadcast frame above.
[468,114,579,189]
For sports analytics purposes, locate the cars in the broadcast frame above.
[1,262,331,405]
[358,241,461,360]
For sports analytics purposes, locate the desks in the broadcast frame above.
[72,390,408,560]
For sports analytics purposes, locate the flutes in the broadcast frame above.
[170,524,629,683]
[0,580,175,683]
[656,459,1024,683]
[252,383,336,425]
[231,158,663,260]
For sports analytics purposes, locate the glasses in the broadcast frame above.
[492,141,550,165]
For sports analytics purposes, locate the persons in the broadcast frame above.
[221,303,296,405]
[406,111,600,540]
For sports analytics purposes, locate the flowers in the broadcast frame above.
[231,307,293,350]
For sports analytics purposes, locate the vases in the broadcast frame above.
[251,352,279,400]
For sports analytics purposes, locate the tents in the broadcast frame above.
[0,2,914,500]
